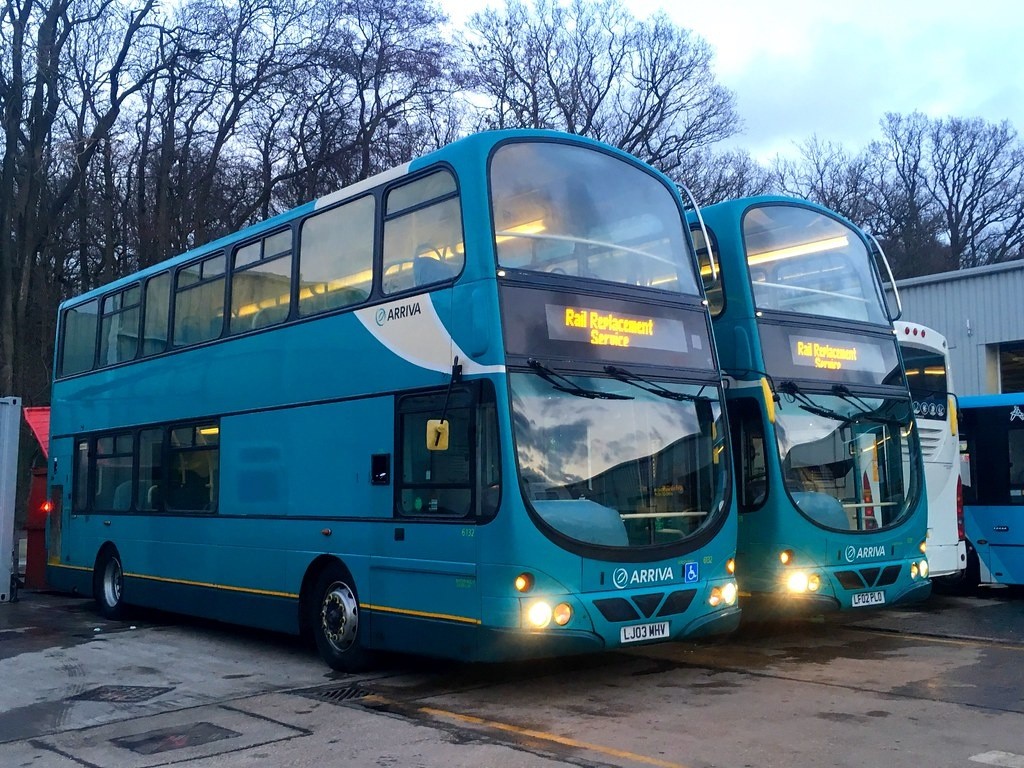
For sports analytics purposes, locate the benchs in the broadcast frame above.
[114,244,446,365]
[78,462,200,511]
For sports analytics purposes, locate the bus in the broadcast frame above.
[505,194,1024,628]
[44,129,740,670]
